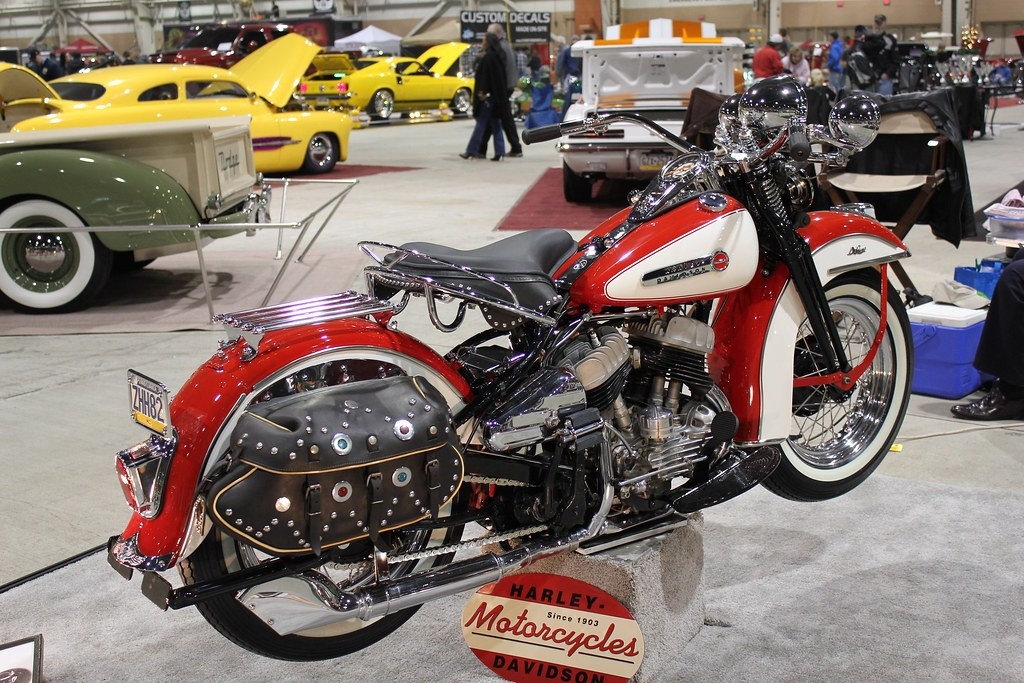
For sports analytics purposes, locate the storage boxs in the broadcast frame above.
[986,214,1024,240]
[905,300,991,402]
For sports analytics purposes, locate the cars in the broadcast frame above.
[297,42,474,115]
[0,32,359,177]
[0,125,264,309]
[558,17,751,206]
[117,20,330,75]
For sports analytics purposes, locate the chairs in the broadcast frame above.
[816,84,956,298]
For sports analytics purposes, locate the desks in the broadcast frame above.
[978,82,1024,137]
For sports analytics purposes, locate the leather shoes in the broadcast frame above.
[950,377,1024,420]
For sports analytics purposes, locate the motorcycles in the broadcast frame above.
[108,76,915,665]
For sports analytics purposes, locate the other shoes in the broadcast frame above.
[474,152,486,159]
[504,151,523,157]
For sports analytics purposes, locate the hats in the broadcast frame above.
[853,25,868,37]
[770,34,787,47]
[573,35,580,40]
[829,31,838,39]
[999,58,1006,61]
[875,15,886,24]
[514,47,529,51]
[30,49,39,60]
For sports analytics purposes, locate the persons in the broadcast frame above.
[743,12,1013,104]
[459,23,592,160]
[951,246,1024,421]
[28,47,135,81]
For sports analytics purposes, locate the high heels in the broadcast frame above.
[459,153,475,160]
[491,154,505,161]
